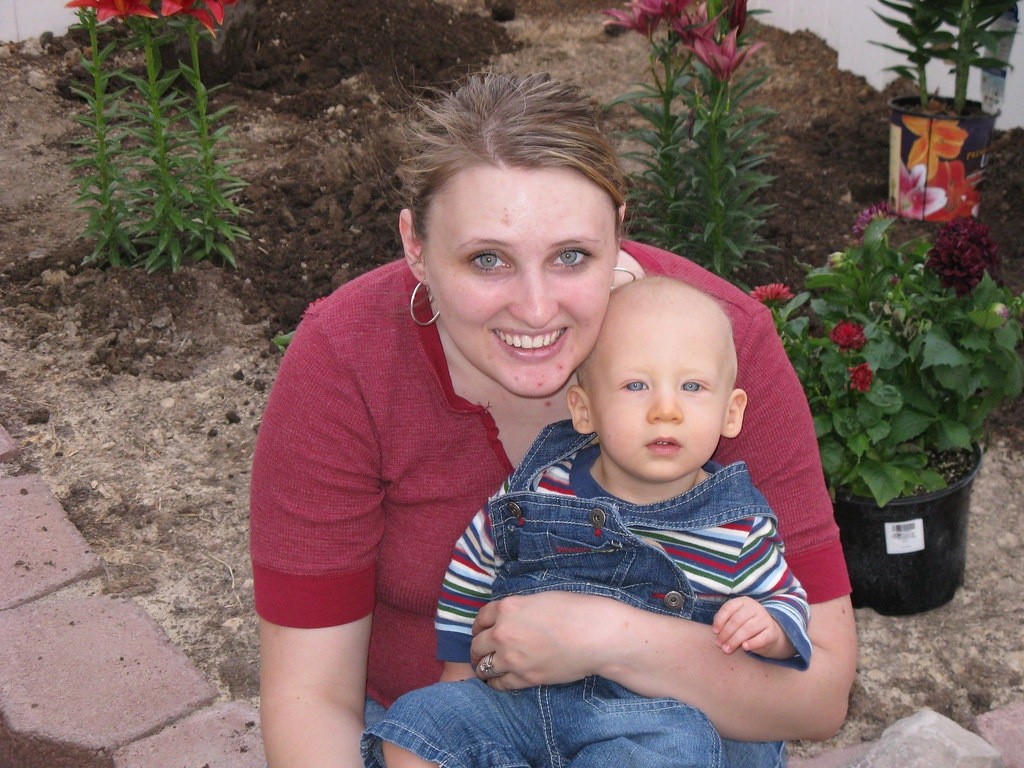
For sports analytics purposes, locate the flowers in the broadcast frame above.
[745,202,1024,507]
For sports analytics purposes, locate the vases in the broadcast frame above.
[840,441,983,616]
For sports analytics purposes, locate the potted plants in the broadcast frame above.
[865,0,1020,174]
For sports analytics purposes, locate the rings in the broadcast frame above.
[480,653,504,677]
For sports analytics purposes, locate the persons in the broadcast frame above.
[360,277,812,768]
[249,73,857,768]
